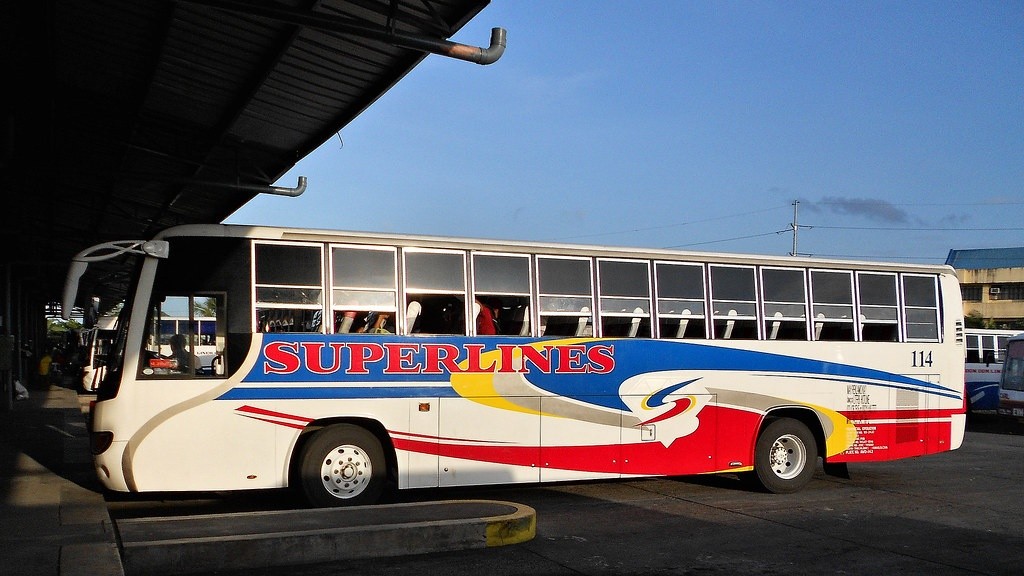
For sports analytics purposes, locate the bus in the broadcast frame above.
[996,333,1024,426]
[73,315,222,393]
[965,328,1024,413]
[58,224,968,496]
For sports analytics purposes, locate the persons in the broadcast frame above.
[166,334,202,376]
[302,285,504,335]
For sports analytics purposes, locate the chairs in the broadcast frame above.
[314,300,866,342]
